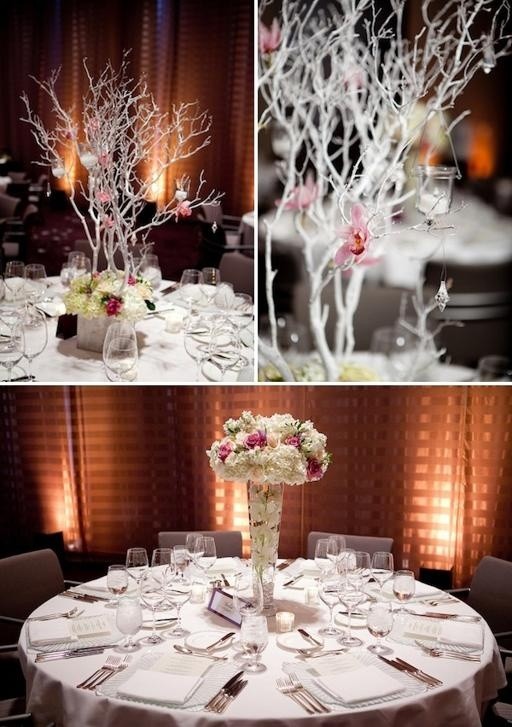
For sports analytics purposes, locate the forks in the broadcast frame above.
[416,640,483,664]
[276,669,332,718]
[78,652,134,692]
[422,597,459,608]
[28,607,88,624]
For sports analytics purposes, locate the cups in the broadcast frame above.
[258,311,445,383]
[414,162,454,216]
[276,613,295,632]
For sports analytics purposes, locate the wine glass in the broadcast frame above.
[309,530,416,655]
[0,256,254,386]
[106,531,221,651]
[227,566,270,673]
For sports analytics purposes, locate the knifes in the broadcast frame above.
[409,609,488,625]
[35,644,107,663]
[207,632,235,648]
[205,672,248,714]
[377,654,447,689]
[56,579,109,605]
[298,629,320,646]
[220,573,230,588]
[275,552,305,589]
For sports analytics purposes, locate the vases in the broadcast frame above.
[246,475,279,612]
[78,314,137,352]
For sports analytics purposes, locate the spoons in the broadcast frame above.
[296,648,348,658]
[173,644,227,661]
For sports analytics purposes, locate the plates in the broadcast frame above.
[279,629,321,652]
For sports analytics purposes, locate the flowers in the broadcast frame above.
[208,412,332,610]
[63,267,155,320]
